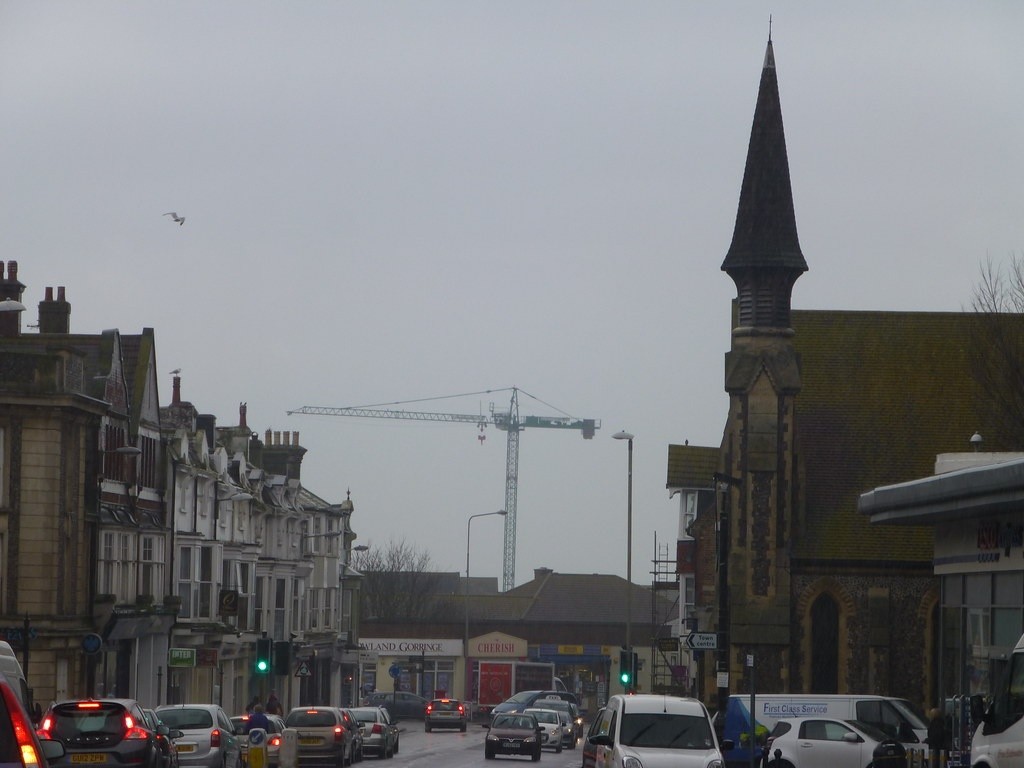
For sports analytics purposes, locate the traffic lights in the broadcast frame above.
[254,637,272,675]
[619,651,631,689]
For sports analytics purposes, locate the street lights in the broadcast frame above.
[462,509,509,703]
[611,431,634,694]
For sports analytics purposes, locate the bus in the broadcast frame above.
[721,692,951,768]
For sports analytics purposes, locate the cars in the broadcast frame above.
[486,690,584,754]
[760,715,908,768]
[424,697,467,733]
[362,690,432,722]
[482,712,546,763]
[345,703,400,760]
[153,702,244,768]
[228,713,288,768]
[279,705,366,768]
[0,638,185,768]
[581,706,607,768]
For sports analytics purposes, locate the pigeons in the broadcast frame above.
[685,439,688,446]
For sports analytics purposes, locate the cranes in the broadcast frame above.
[285,384,603,595]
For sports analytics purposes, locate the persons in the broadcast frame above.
[244,691,283,734]
[873,739,906,768]
[923,709,945,768]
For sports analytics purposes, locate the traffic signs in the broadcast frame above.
[686,631,718,651]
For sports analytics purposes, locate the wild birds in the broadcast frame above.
[169,368,181,376]
[244,401,247,405]
[162,212,185,226]
[240,401,242,405]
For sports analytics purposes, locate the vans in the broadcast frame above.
[588,692,735,768]
[968,633,1024,768]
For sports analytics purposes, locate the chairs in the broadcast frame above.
[162,716,176,726]
[547,715,553,723]
[201,716,211,725]
[521,720,530,728]
